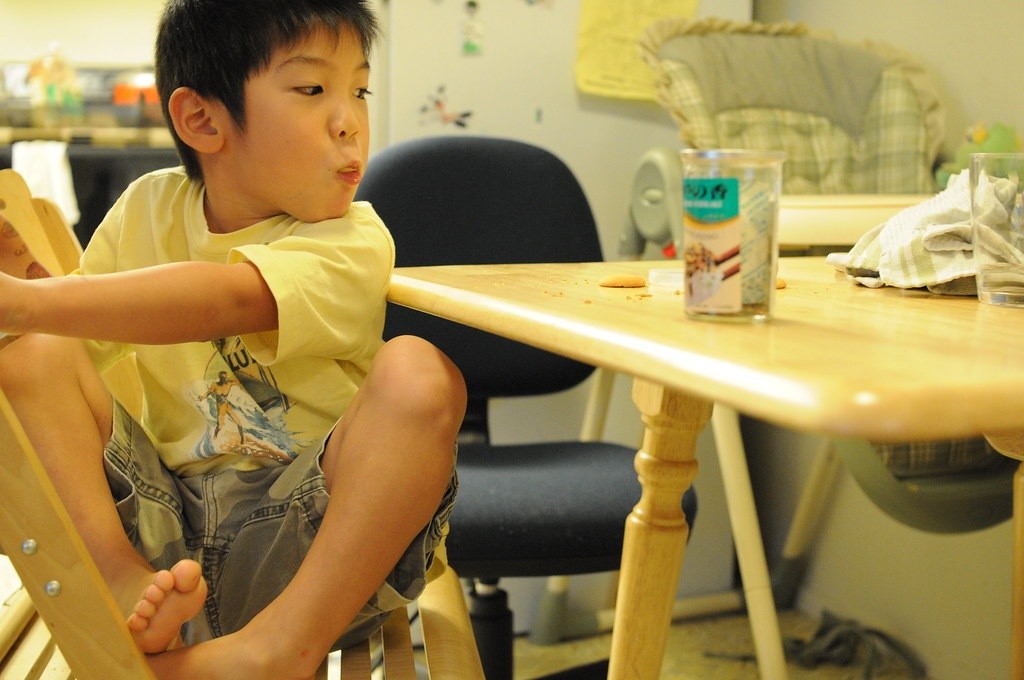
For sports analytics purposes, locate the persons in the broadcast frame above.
[1,0,468,680]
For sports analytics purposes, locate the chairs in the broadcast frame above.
[0,134,697,680]
[527,17,1014,680]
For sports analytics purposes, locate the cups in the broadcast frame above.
[680,149,784,324]
[969,152,1024,308]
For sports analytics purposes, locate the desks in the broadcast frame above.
[386,257,1024,680]
